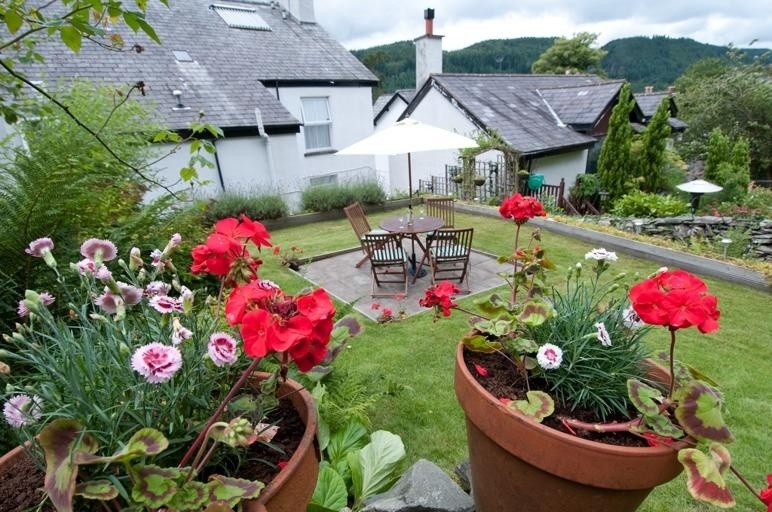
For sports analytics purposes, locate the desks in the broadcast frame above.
[377,213,445,270]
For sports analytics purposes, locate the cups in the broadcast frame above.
[398,216,404,228]
[419,207,425,220]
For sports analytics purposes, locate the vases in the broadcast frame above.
[452,328,700,510]
[0,371,319,512]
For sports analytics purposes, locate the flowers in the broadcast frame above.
[420,191,772,511]
[0,211,335,512]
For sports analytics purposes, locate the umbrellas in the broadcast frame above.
[332,117,480,218]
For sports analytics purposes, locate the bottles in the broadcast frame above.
[407,204,414,225]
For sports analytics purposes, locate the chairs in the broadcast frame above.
[410,227,474,295]
[364,232,408,300]
[343,201,412,269]
[424,195,456,265]
